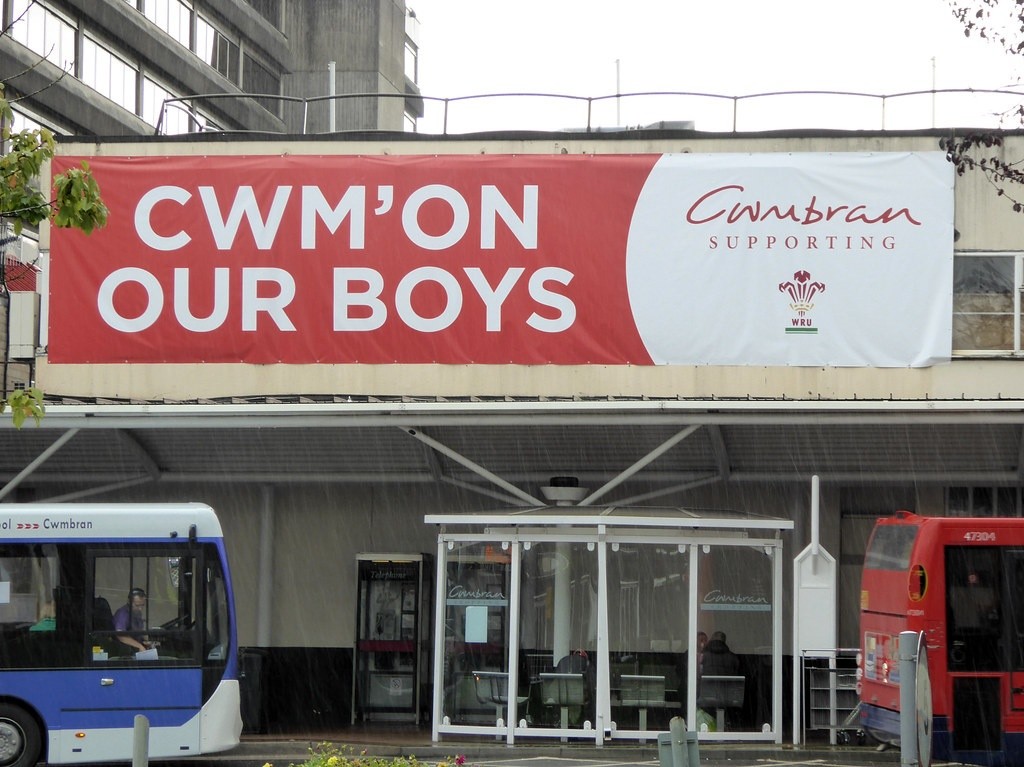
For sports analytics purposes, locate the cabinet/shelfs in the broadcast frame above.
[810,669,860,731]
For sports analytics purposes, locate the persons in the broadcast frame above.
[556,648,594,701]
[29,604,56,630]
[674,631,739,710]
[112,589,146,652]
[614,651,643,699]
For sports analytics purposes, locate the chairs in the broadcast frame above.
[619,674,665,744]
[610,661,682,708]
[472,670,529,740]
[93,596,140,658]
[540,673,585,743]
[697,675,745,743]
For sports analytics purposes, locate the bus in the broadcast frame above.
[0,502,244,766]
[855,510,1024,767]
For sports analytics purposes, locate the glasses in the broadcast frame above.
[698,639,707,642]
